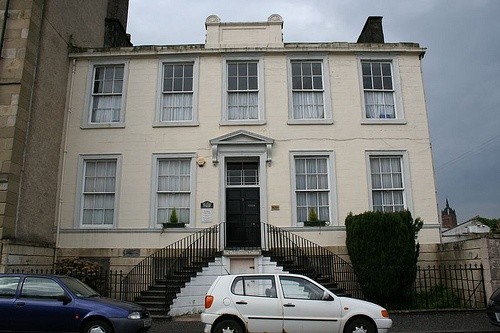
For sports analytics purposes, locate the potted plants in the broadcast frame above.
[162,206,186,228]
[303,207,326,227]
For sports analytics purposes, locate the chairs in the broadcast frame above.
[265,288,271,296]
[270,287,277,297]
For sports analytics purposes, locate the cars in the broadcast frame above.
[0,273,152,333]
[200,274,393,333]
